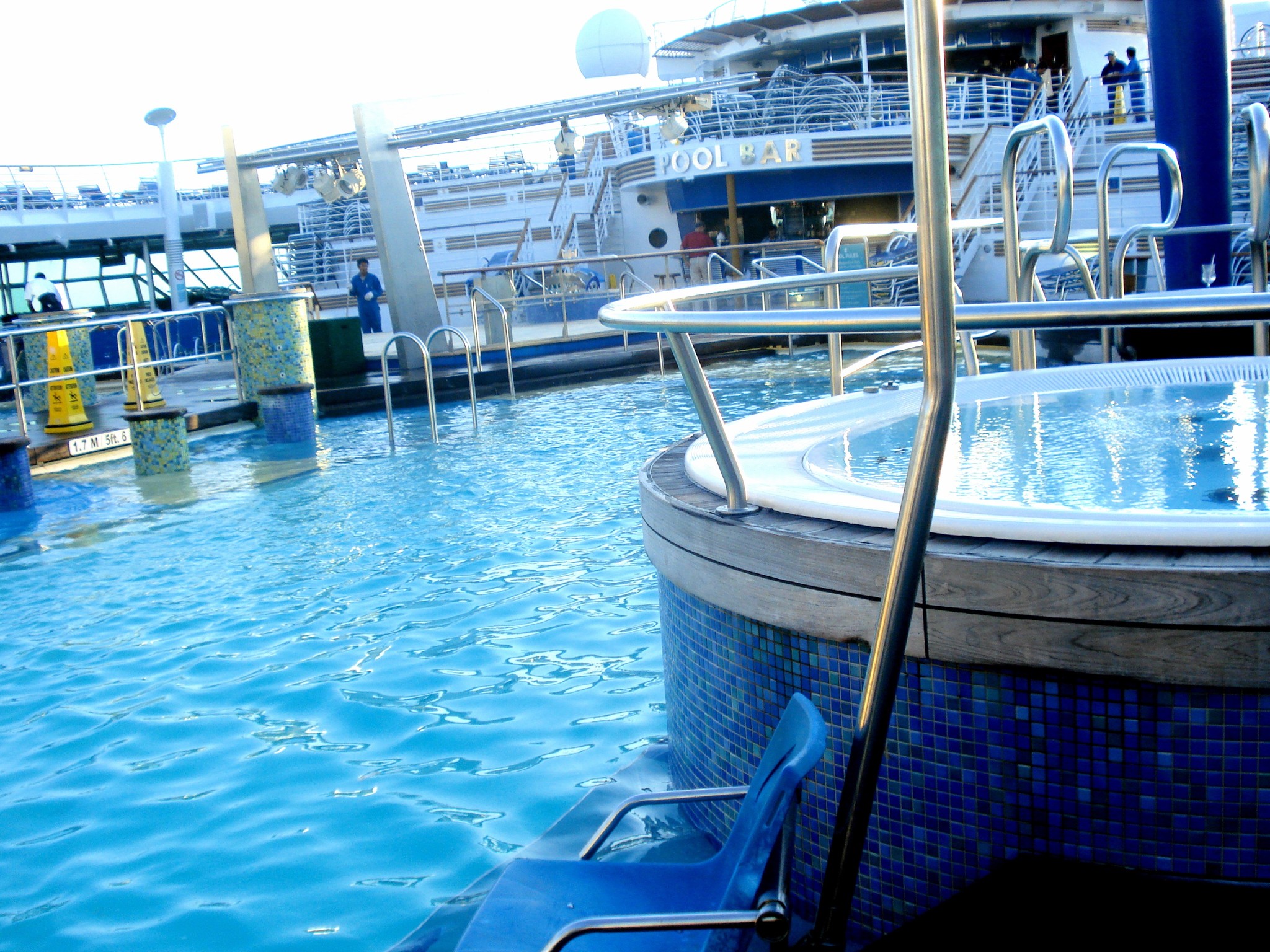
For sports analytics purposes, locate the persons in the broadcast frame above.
[870,55,1054,127]
[760,224,786,243]
[1101,47,1147,123]
[680,222,718,311]
[25,272,64,314]
[554,120,580,180]
[346,258,383,334]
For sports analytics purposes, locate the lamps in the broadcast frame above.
[269,155,366,204]
[553,118,584,155]
[660,93,713,140]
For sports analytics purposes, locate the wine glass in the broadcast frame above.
[1201,264,1216,288]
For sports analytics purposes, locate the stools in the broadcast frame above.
[685,274,694,285]
[668,273,681,287]
[655,274,666,288]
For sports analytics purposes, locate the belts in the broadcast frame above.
[689,254,710,259]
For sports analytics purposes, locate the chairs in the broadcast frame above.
[463,249,600,305]
[871,216,1270,306]
[454,691,826,952]
[287,198,374,291]
[405,148,535,185]
[681,62,975,142]
[89,300,227,375]
[0,177,228,211]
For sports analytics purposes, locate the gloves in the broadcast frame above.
[365,291,373,301]
[346,282,353,290]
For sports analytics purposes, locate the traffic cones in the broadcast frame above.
[125,322,166,412]
[43,330,104,434]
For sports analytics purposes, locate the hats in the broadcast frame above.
[694,222,706,228]
[1104,49,1118,57]
